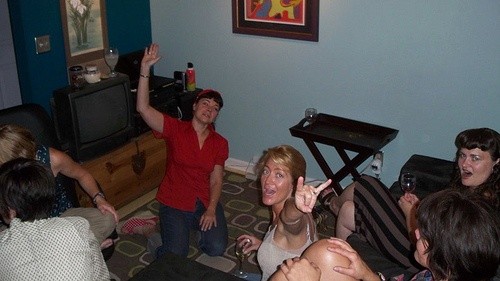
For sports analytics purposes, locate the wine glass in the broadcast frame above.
[104,47,119,77]
[400,173,417,205]
[233,238,252,279]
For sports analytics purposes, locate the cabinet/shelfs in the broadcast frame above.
[72,80,207,216]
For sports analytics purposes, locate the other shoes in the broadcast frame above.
[314,183,337,220]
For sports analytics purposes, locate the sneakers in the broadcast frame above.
[115,216,161,236]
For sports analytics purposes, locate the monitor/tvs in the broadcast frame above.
[52,72,133,163]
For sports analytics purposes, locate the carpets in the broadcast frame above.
[105,171,335,281]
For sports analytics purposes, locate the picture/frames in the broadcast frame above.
[59,0,108,65]
[232,0,319,42]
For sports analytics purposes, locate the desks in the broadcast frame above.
[289,113,399,194]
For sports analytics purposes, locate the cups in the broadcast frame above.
[305,108,317,123]
[69,66,84,88]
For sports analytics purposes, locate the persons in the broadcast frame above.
[0,125,119,262]
[270,188,500,281]
[0,157,110,281]
[237,145,332,281]
[318,127,500,273]
[120,43,229,259]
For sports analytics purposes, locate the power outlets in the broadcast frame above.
[371,152,383,171]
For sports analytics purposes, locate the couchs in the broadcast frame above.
[392,154,456,200]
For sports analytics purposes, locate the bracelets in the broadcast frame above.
[140,74,149,78]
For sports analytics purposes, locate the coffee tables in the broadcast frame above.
[128,252,246,281]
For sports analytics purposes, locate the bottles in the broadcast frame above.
[186,62,196,91]
[83,64,101,84]
